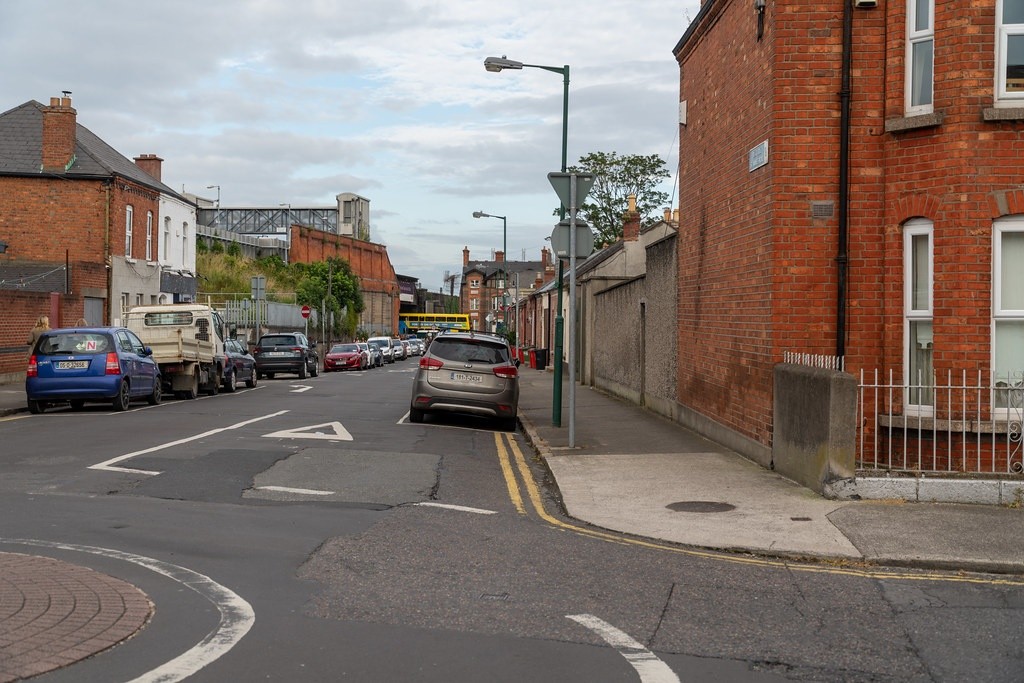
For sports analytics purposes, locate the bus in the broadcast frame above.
[399,313,473,339]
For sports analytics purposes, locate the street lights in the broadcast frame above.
[484,54,571,429]
[472,211,507,334]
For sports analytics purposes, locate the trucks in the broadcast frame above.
[125,303,239,402]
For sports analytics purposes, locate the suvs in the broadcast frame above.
[223,337,258,393]
[410,329,518,433]
[254,331,320,380]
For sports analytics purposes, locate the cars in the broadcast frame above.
[324,336,426,371]
[24,326,162,415]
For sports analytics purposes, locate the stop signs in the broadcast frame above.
[301,306,311,319]
